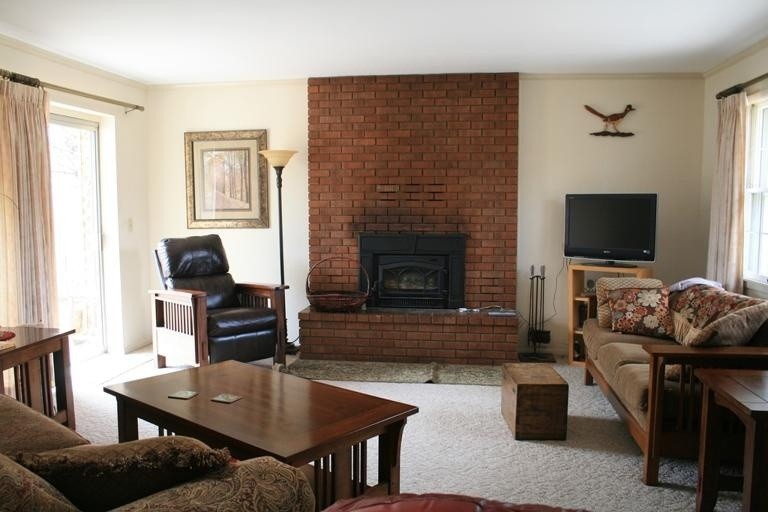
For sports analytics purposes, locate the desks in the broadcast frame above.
[0,322,78,434]
[691,365,767,511]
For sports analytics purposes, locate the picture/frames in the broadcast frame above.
[182,127,270,232]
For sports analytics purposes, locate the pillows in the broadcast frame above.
[663,276,765,348]
[604,284,673,340]
[11,434,232,511]
[592,275,663,329]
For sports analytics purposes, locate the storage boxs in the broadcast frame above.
[497,361,569,444]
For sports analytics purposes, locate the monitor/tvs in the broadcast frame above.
[564,193,659,267]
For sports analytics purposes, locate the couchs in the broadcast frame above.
[149,232,289,367]
[0,394,316,512]
[580,282,766,485]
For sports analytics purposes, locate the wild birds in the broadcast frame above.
[584,105,636,133]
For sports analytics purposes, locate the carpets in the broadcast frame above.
[56,357,744,511]
[281,356,510,389]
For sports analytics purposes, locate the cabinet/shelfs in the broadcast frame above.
[564,264,651,368]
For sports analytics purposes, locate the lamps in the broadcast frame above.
[517,264,559,363]
[255,148,298,354]
